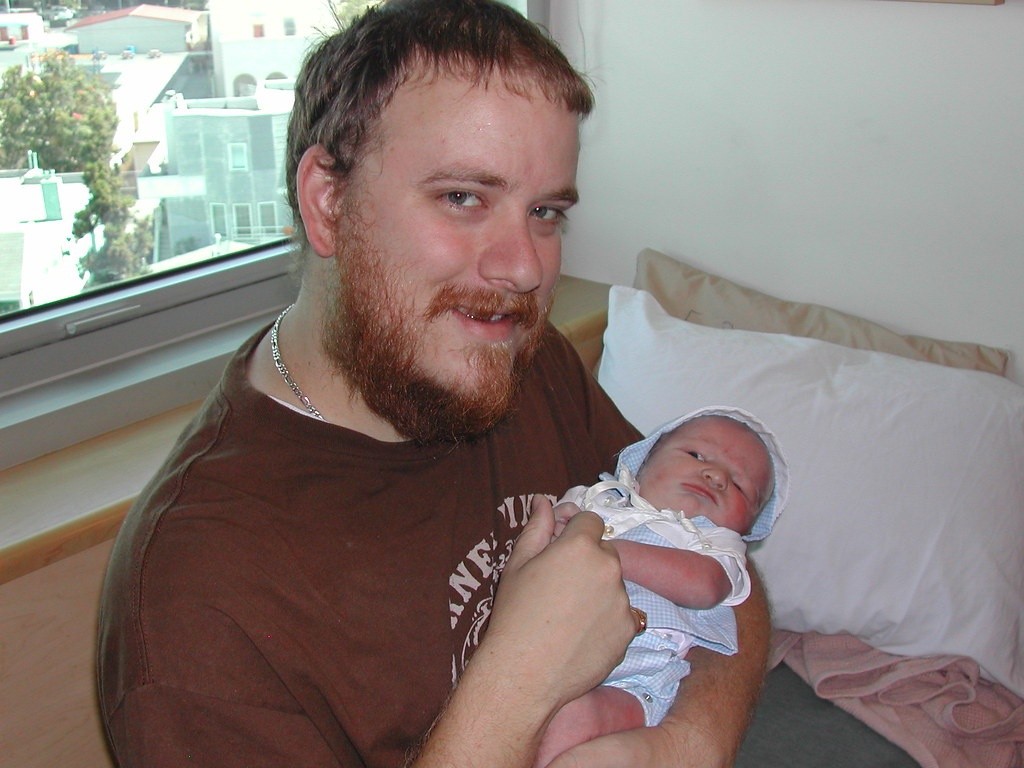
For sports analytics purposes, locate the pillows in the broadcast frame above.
[594,285,1023,695]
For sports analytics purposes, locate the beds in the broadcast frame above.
[0,250,1023,767]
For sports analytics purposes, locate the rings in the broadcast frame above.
[630,607,647,637]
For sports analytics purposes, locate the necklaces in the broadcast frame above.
[271,304,324,421]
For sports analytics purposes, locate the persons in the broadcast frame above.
[530,405,790,768]
[95,0,771,768]
